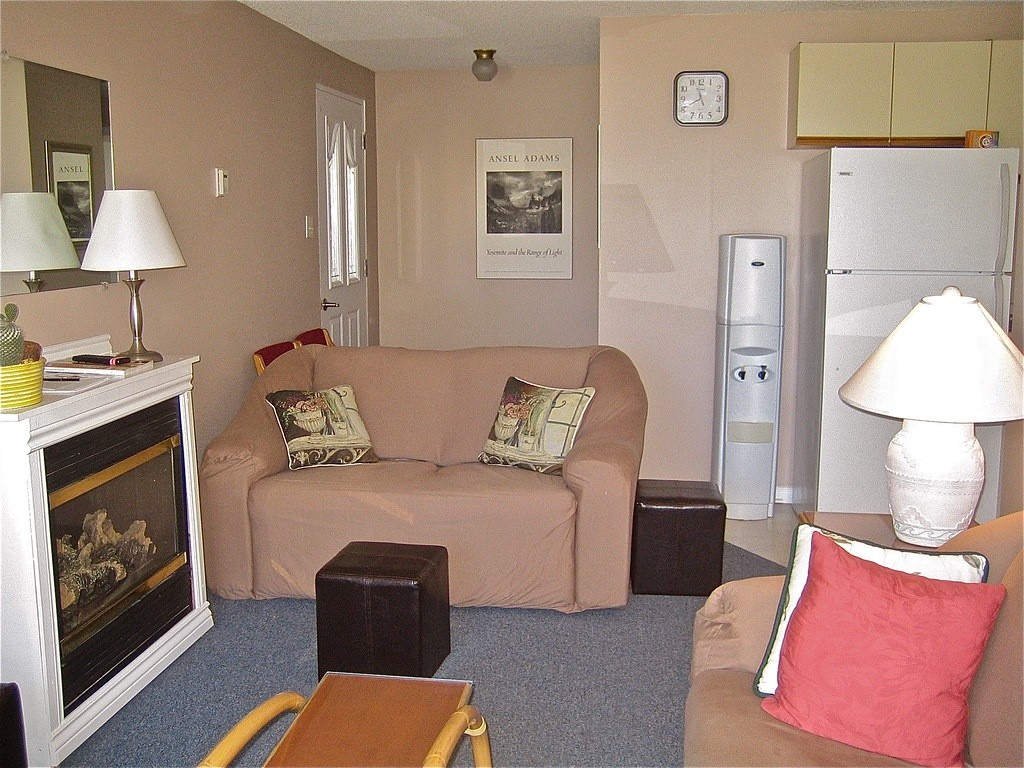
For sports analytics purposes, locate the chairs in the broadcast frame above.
[294,327,334,345]
[253,341,297,375]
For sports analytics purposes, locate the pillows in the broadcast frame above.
[476,375,595,476]
[753,523,989,697]
[264,383,379,471]
[761,531,1007,768]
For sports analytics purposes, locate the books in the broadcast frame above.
[44,354,154,377]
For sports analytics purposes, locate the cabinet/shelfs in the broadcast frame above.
[787,39,1023,169]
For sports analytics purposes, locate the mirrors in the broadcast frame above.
[0,49,120,298]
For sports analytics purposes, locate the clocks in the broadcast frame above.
[673,70,729,127]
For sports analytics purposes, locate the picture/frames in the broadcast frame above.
[45,140,95,246]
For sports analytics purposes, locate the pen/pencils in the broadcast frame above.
[43,378,80,381]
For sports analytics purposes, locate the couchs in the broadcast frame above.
[683,511,1024,768]
[199,344,648,614]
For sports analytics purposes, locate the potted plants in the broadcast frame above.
[0,302,47,410]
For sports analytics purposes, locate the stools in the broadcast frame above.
[314,542,451,683]
[629,480,727,597]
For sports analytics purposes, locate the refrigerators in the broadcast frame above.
[790,147,1019,526]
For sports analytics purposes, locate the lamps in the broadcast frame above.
[0,192,81,294]
[80,189,188,363]
[838,286,1024,547]
[471,48,498,82]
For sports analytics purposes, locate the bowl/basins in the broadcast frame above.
[0,355,46,410]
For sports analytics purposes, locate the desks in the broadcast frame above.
[195,671,493,768]
[0,355,214,768]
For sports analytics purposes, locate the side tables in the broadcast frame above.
[797,510,979,552]
